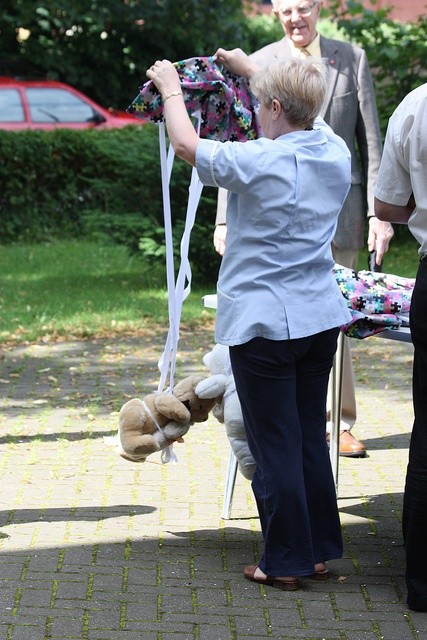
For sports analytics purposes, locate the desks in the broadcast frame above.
[200,280,412,519]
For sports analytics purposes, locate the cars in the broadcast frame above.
[0,79,148,130]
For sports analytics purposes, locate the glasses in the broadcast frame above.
[281,2,318,17]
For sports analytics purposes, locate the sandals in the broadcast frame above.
[314,561,328,580]
[244,566,299,591]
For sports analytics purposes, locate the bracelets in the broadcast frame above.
[162,92,182,105]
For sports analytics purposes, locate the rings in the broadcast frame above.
[151,66,154,72]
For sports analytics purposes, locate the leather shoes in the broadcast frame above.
[338,430,366,457]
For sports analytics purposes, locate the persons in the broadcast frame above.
[213,0,393,459]
[146,48,353,593]
[373,77,426,614]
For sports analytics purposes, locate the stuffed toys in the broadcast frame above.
[117,373,218,466]
[195,343,260,482]
